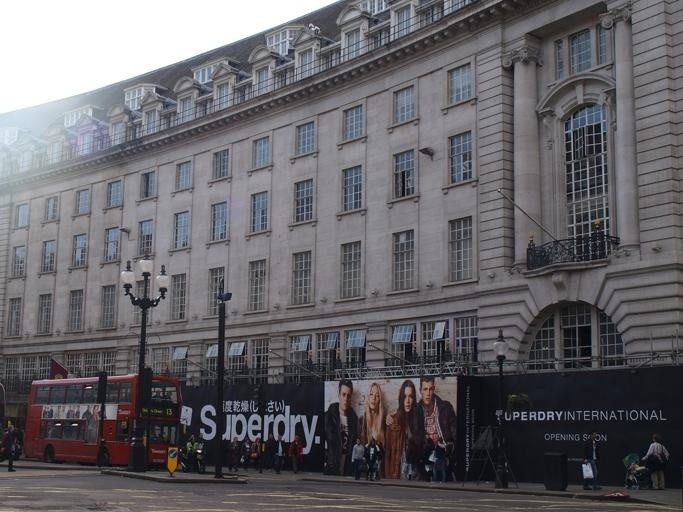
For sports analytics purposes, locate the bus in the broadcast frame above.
[22,374,183,471]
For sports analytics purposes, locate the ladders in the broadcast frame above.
[462,426,519,489]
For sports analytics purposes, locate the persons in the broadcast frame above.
[184,432,206,473]
[151,389,162,399]
[241,437,253,468]
[41,405,81,419]
[380,379,421,479]
[229,437,242,472]
[580,430,602,491]
[364,438,379,478]
[162,391,173,401]
[640,433,671,490]
[87,405,100,444]
[354,381,389,479]
[0,424,25,472]
[275,434,286,474]
[445,436,456,481]
[422,436,435,481]
[288,435,303,473]
[384,377,458,482]
[352,435,364,480]
[629,458,643,478]
[81,404,92,421]
[251,437,264,473]
[264,432,277,471]
[433,436,446,481]
[321,378,357,477]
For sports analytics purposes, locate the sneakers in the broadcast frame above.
[583,484,601,490]
[7,468,15,472]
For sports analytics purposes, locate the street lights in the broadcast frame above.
[120,248,170,472]
[493,327,509,489]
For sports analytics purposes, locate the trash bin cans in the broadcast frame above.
[543,452,568,491]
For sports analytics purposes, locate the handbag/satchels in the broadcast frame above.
[250,452,258,459]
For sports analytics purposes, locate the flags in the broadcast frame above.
[48,355,69,380]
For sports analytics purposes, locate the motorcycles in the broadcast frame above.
[180,447,207,474]
